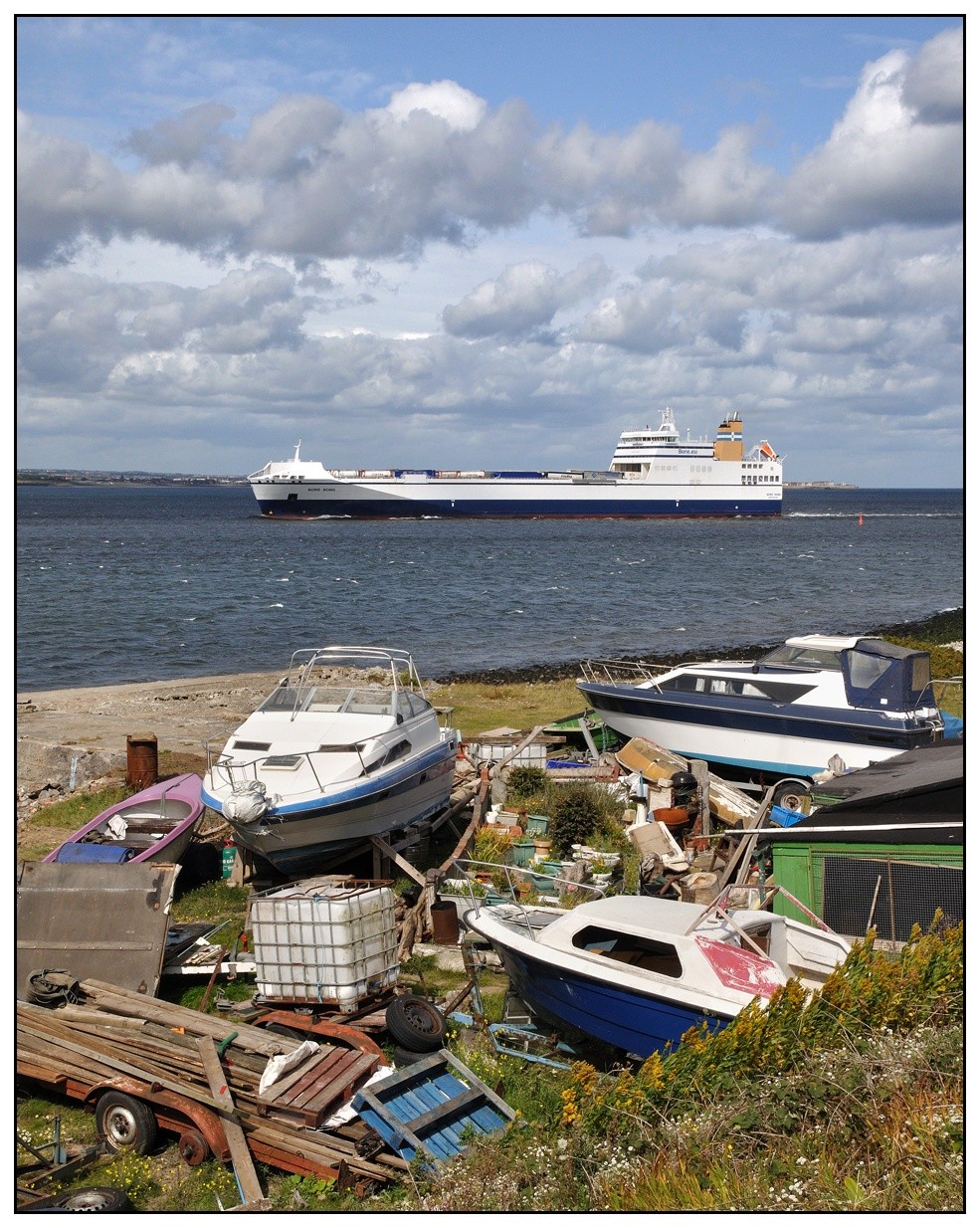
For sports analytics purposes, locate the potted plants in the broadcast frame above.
[587,835,620,868]
[464,801,536,901]
[437,869,488,932]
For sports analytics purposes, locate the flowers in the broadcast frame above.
[525,799,612,874]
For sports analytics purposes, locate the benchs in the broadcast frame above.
[256,1043,380,1127]
[106,818,184,833]
[103,840,161,856]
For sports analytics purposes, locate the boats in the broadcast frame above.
[247,406,788,520]
[575,633,964,779]
[541,709,619,752]
[198,645,459,872]
[760,445,775,461]
[40,772,207,865]
[453,857,853,1069]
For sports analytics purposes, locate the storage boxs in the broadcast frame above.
[643,884,681,901]
[629,821,672,862]
[768,805,808,827]
[546,760,590,769]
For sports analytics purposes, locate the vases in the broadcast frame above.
[561,861,574,872]
[534,839,551,855]
[539,858,563,872]
[593,872,612,880]
[527,814,550,834]
[529,866,559,890]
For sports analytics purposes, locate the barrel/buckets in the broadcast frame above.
[760,445,773,458]
[404,835,430,872]
[55,841,134,863]
[430,900,459,947]
[127,735,158,791]
